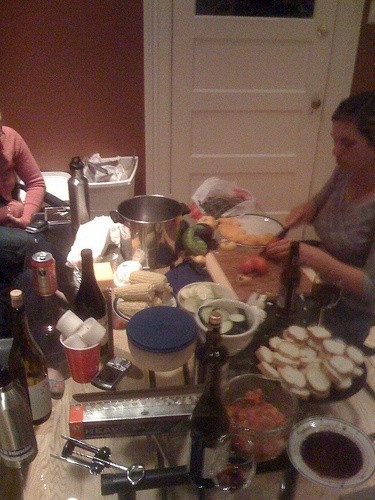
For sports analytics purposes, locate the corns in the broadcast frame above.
[113,270,172,315]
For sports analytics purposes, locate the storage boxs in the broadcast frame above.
[81,157,139,212]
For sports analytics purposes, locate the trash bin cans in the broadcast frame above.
[83,154,140,219]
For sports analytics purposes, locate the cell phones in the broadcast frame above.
[91,356,132,390]
[48,379,65,398]
[25,219,48,233]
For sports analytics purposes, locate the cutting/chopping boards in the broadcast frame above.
[214,247,286,303]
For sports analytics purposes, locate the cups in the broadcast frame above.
[59,333,101,385]
[217,373,296,463]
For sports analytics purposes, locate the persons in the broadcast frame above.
[264,91,375,347]
[0,119,47,293]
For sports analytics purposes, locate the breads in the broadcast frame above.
[255,324,364,401]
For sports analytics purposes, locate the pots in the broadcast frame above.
[109,194,191,269]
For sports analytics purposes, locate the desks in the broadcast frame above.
[1,251,375,500]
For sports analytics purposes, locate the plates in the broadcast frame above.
[286,414,375,488]
[111,296,178,322]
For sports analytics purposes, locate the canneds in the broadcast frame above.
[31,251,58,296]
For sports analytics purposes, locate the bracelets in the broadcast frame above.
[5,205,14,216]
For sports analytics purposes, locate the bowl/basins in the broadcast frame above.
[194,299,259,357]
[250,321,367,403]
[217,212,287,250]
[175,280,234,315]
[126,305,199,373]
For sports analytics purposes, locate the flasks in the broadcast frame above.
[68,156,90,230]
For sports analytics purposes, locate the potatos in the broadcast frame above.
[197,215,276,250]
[240,255,269,274]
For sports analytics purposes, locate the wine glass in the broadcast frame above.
[309,271,343,327]
[210,433,257,500]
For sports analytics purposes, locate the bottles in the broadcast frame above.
[198,311,228,384]
[0,364,38,470]
[73,248,109,356]
[8,289,53,425]
[188,347,229,489]
[274,240,301,318]
